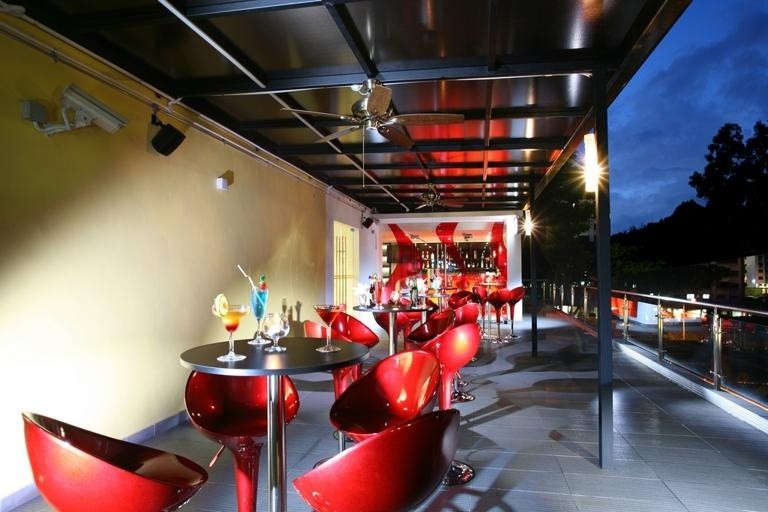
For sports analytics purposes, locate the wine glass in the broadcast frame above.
[312,305,343,352]
[217,305,247,362]
[262,313,291,353]
[247,286,272,345]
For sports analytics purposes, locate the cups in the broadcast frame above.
[441,288,446,295]
[359,295,367,307]
[417,297,425,305]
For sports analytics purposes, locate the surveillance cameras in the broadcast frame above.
[60,85,129,134]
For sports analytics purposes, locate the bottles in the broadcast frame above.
[410,280,418,306]
[421,250,458,267]
[369,280,382,309]
[460,249,490,268]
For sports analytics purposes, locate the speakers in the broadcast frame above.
[151,123,185,156]
[362,218,373,228]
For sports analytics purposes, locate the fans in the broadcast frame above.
[414,182,470,210]
[279,78,465,151]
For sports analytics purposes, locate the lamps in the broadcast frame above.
[149,112,186,157]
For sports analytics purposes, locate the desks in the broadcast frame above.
[178,337,364,512]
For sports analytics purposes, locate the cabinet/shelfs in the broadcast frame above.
[381,241,499,278]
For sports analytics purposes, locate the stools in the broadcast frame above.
[294,408,458,511]
[303,285,525,485]
[20,410,208,512]
[186,370,300,511]
